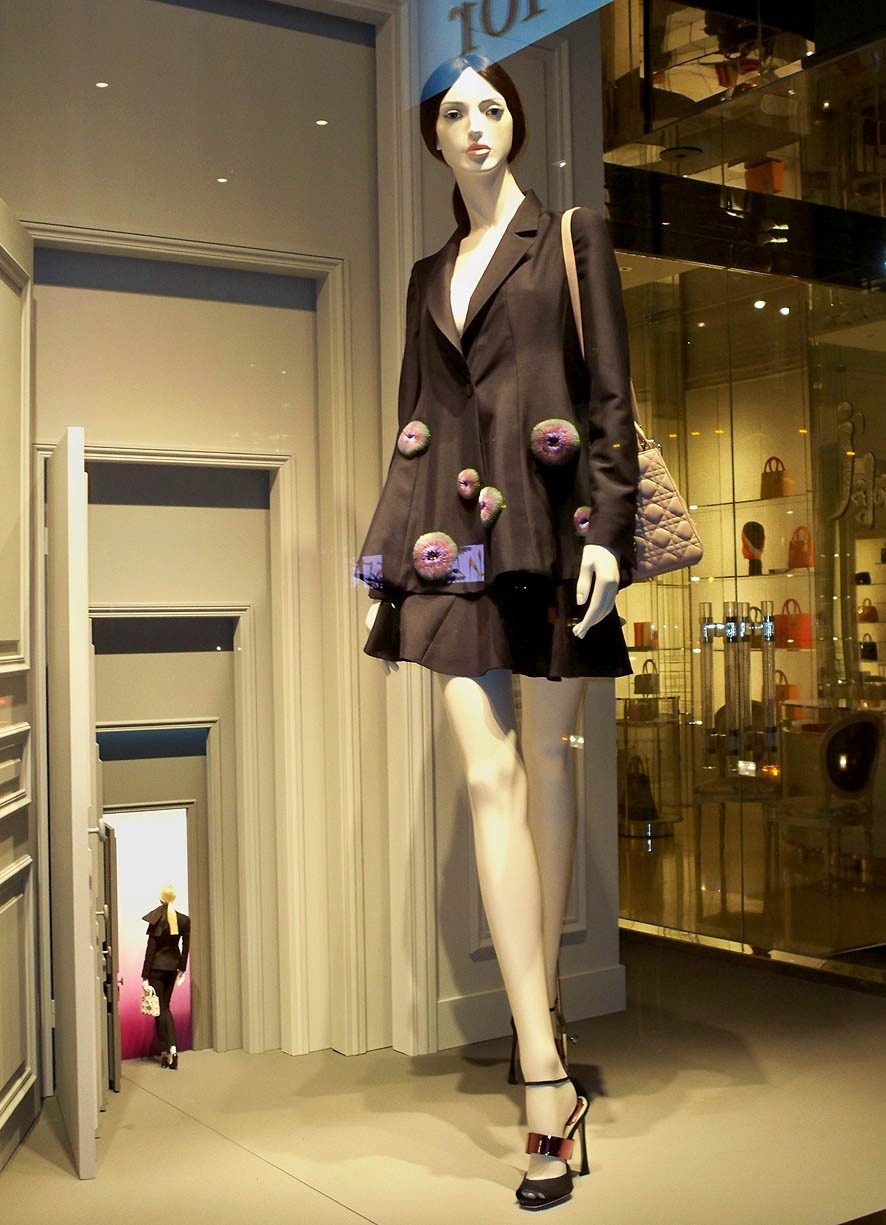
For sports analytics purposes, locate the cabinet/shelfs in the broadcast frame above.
[612,488,886,736]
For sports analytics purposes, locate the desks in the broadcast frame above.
[782,719,886,872]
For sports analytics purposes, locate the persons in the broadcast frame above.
[140,883,190,1069]
[352,53,640,1211]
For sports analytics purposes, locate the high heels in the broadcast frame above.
[516,1079,589,1209]
[508,996,587,1097]
[161,1053,178,1070]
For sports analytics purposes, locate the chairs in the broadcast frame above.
[763,711,880,903]
[692,727,798,890]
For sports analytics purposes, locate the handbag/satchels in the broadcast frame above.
[854,572,879,661]
[761,458,794,499]
[558,204,705,590]
[788,527,813,571]
[620,756,661,825]
[880,543,886,564]
[625,658,662,721]
[141,987,161,1018]
[760,669,805,720]
[750,600,815,649]
